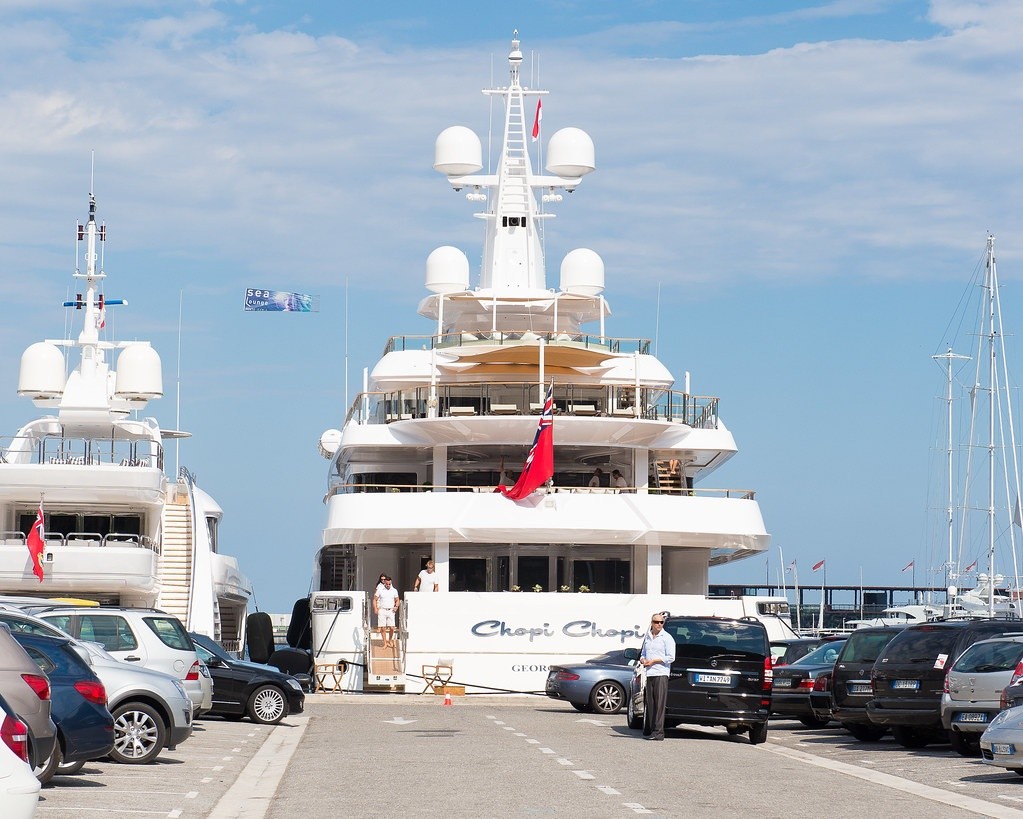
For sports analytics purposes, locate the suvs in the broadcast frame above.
[827,622,919,744]
[624,610,775,743]
[866,615,1023,749]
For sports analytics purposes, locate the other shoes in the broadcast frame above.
[382,645,387,650]
[389,642,394,648]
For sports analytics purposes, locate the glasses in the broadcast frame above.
[381,579,387,581]
[652,620,665,624]
[427,566,434,569]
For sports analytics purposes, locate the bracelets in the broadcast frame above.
[415,586,418,588]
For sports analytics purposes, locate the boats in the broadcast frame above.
[1,143,255,663]
[303,25,772,698]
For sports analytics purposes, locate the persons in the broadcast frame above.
[414,561,438,592]
[589,468,602,487]
[373,577,400,647]
[669,460,678,474]
[376,573,387,632]
[612,470,629,492]
[641,614,675,739]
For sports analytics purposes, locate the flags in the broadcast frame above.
[498,384,552,501]
[902,560,912,572]
[785,560,794,573]
[27,502,44,583]
[532,99,542,141]
[966,562,976,571]
[812,560,824,573]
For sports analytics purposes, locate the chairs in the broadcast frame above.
[385,403,636,423]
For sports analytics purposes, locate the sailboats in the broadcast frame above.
[770,231,1023,639]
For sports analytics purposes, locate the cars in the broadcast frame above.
[939,629,1023,757]
[82,630,306,725]
[977,657,1023,777]
[1,591,214,819]
[767,633,821,665]
[544,648,642,716]
[771,640,848,729]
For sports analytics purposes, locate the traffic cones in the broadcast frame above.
[443,689,453,706]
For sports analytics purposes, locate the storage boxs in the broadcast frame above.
[433,686,465,695]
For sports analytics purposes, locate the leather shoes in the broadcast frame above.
[642,734,658,740]
